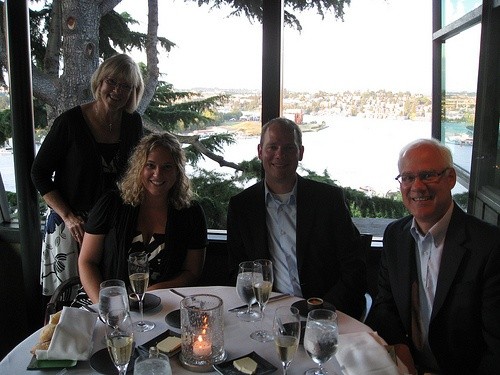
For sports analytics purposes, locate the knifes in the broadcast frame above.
[228,293,291,312]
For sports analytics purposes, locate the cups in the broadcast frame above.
[133,352,173,375]
[180,293,227,372]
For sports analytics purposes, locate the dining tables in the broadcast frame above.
[0,285,412,375]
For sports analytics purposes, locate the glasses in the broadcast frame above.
[395,167,449,185]
[104,78,133,92]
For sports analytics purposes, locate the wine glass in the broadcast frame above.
[127,252,156,333]
[105,309,133,375]
[98,279,130,335]
[303,309,339,375]
[248,260,277,342]
[273,306,301,375]
[236,261,264,323]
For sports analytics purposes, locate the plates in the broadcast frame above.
[89,346,141,375]
[128,293,160,311]
[290,299,337,319]
[165,308,180,329]
[137,329,182,358]
[212,351,278,375]
[27,355,78,369]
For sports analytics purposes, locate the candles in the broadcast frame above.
[193,335,212,357]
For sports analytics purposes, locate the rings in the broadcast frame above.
[75,232,80,236]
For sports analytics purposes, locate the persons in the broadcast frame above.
[69,133,209,310]
[32,54,144,296]
[226,117,371,323]
[373,139,500,375]
